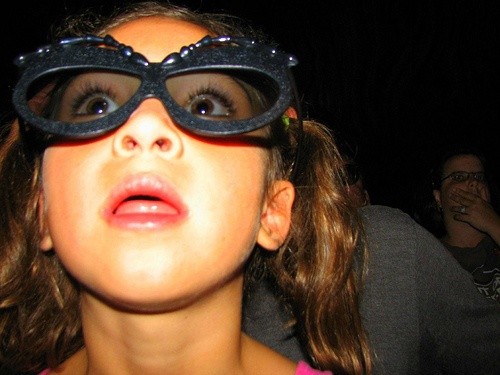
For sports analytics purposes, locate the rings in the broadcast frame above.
[460,205,467,214]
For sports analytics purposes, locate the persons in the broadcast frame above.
[240,200,499,375]
[430,144,500,306]
[328,135,371,207]
[0,3,373,375]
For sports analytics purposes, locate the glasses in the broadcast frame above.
[9,32,296,137]
[441,170,484,185]
